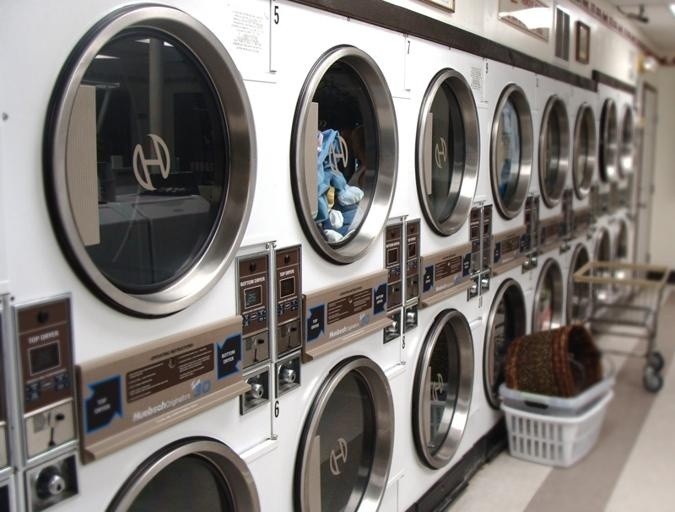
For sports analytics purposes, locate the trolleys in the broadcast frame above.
[570,258,675,393]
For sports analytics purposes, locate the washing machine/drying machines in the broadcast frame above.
[0,1,643,512]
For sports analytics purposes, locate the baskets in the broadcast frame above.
[498,324,615,467]
